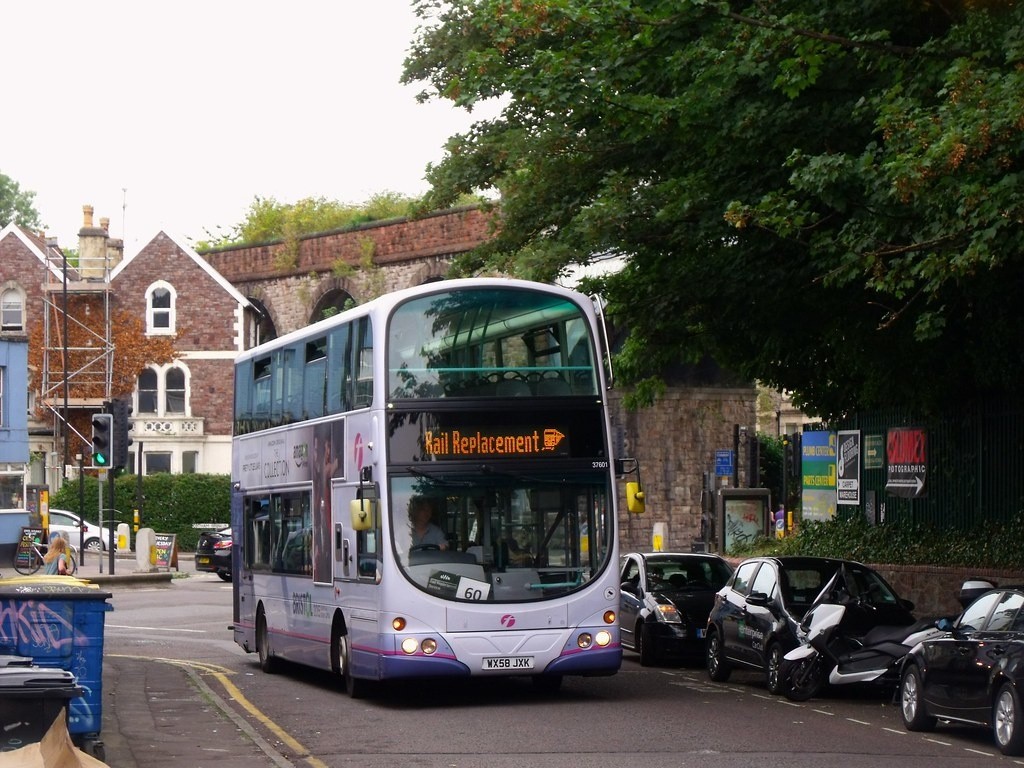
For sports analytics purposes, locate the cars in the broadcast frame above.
[49,509,120,551]
[619,551,737,669]
[895,586,1024,758]
[195,526,232,583]
[703,555,918,697]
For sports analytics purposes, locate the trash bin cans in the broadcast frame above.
[0,655,86,753]
[0,575,115,763]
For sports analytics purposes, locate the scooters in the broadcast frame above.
[778,568,1002,702]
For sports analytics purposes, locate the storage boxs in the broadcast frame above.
[958,577,997,611]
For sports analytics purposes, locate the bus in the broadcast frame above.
[228,276,625,700]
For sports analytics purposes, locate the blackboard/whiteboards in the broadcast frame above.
[153,532,177,568]
[14,526,45,567]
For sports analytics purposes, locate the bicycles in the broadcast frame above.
[12,526,76,576]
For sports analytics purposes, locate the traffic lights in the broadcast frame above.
[92,414,113,470]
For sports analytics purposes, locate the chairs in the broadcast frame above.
[235,369,594,579]
[623,573,843,606]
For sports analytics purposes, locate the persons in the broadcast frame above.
[43,538,73,574]
[311,435,339,582]
[769,503,785,542]
[407,500,449,553]
